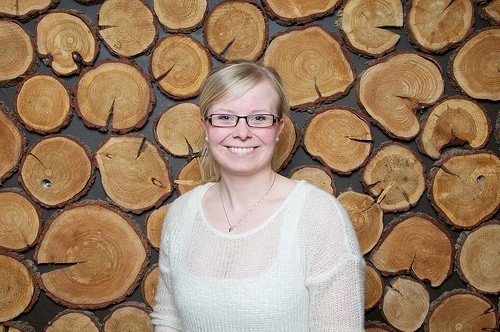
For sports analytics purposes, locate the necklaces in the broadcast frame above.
[218,173,276,232]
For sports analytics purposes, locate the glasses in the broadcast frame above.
[205,114,280,128]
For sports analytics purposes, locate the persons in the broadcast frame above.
[149,59,365,332]
